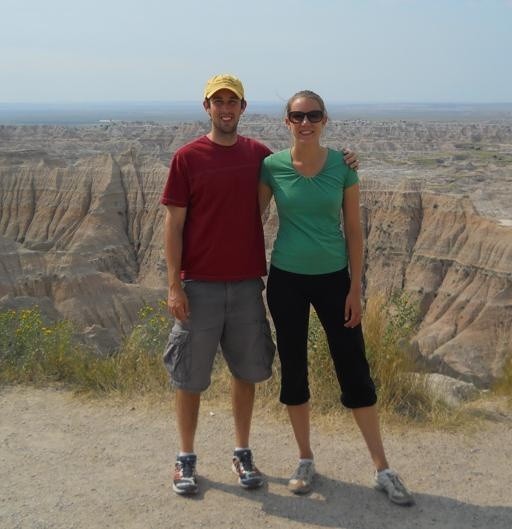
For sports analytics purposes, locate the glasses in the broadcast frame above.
[287,111,324,123]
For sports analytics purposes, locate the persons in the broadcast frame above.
[254,87,418,509]
[157,67,361,499]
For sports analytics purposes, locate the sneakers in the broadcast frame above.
[288,458,314,493]
[231,449,264,489]
[172,450,199,495]
[374,469,415,505]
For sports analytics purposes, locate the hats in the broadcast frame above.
[204,74,244,100]
